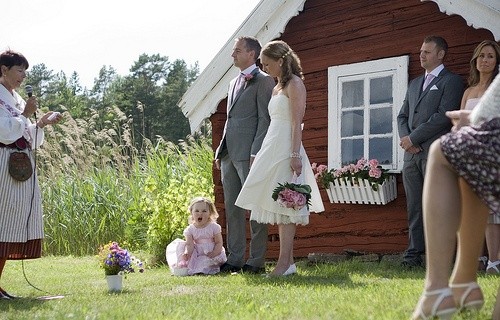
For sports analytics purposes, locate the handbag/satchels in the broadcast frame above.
[8,151,33,181]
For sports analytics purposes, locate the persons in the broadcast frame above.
[397,35,461,271]
[459,39,500,276]
[408,72,500,320]
[214,36,275,275]
[0,47,62,298]
[166,197,228,276]
[258,40,306,276]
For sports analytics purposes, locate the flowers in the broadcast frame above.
[272,171,313,211]
[98,241,144,276]
[243,74,254,89]
[311,158,391,192]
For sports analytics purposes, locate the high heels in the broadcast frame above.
[411,287,454,320]
[449,281,485,320]
[262,264,297,279]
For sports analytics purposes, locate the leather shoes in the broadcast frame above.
[233,263,265,275]
[218,264,244,273]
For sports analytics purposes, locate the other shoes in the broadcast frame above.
[401,260,422,270]
[486,260,500,274]
[478,255,487,272]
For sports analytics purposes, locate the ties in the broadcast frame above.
[423,74,432,92]
[234,73,242,100]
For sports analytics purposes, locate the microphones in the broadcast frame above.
[25,85,36,116]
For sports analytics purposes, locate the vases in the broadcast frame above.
[107,275,122,291]
[325,177,397,205]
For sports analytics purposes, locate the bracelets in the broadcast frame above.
[41,119,47,126]
[290,151,302,158]
